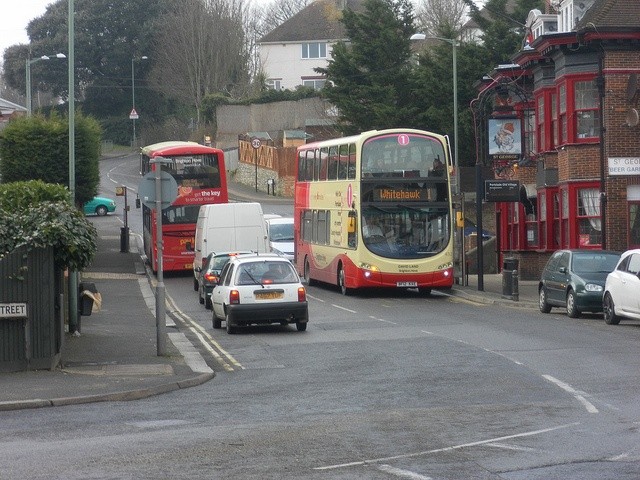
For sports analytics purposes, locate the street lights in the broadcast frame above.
[407,33,461,286]
[25,53,50,121]
[29,52,68,122]
[129,55,150,147]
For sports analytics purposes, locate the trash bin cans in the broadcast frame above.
[502,258,518,296]
[80,282,97,315]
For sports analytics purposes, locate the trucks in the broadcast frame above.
[192,201,269,293]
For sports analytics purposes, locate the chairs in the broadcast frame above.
[421,160,433,171]
[406,161,418,169]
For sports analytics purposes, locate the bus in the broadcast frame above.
[136,140,228,276]
[293,128,456,296]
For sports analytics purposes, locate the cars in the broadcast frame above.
[83,195,116,216]
[537,248,621,318]
[195,250,259,309]
[265,218,296,261]
[210,255,308,334]
[602,249,640,323]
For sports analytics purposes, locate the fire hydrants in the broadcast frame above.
[511,270,520,302]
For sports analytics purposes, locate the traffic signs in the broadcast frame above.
[251,138,262,151]
